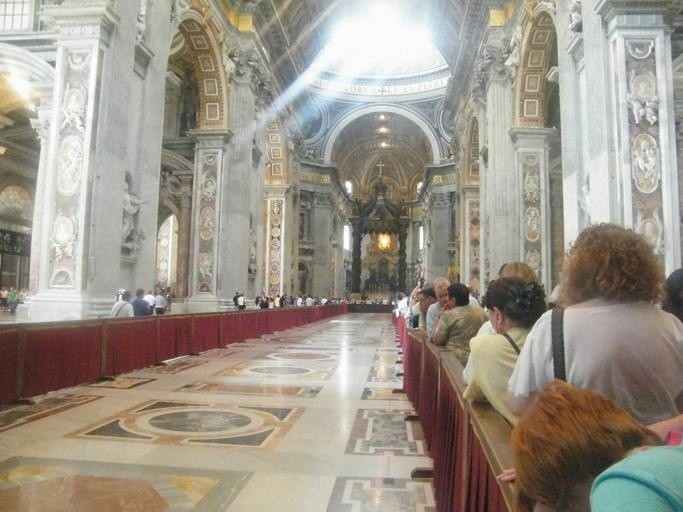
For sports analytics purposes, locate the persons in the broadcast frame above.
[510,380,683,512]
[398,261,560,419]
[121,182,148,240]
[110,288,170,319]
[498,391,682,482]
[508,224,683,424]
[0,285,27,313]
[231,292,397,309]
